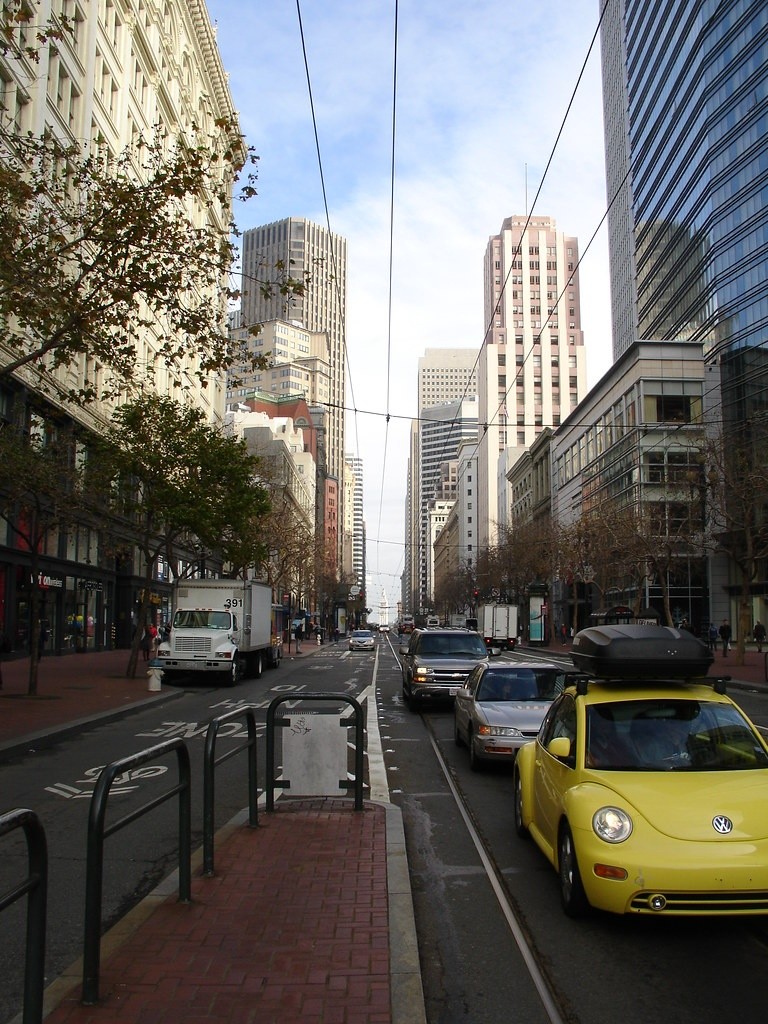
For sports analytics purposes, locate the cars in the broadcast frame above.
[512,623,768,922]
[452,661,582,775]
[349,629,375,650]
[379,625,390,633]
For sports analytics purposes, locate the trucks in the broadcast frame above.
[157,578,284,688]
[448,613,467,630]
[477,603,518,651]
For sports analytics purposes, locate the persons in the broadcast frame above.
[488,680,512,698]
[587,709,697,769]
[398,626,403,644]
[305,621,339,644]
[295,623,303,654]
[561,624,574,646]
[680,620,766,656]
[141,622,171,661]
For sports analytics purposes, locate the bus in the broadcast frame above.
[427,615,440,628]
[399,617,414,633]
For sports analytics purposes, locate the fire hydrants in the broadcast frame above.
[146,658,165,692]
[317,634,321,645]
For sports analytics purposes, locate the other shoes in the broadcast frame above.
[296,651,302,654]
[561,643,566,646]
[400,643,402,644]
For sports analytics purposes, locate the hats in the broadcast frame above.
[149,624,154,626]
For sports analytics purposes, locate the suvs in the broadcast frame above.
[399,626,502,712]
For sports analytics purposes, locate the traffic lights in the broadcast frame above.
[474,591,478,595]
